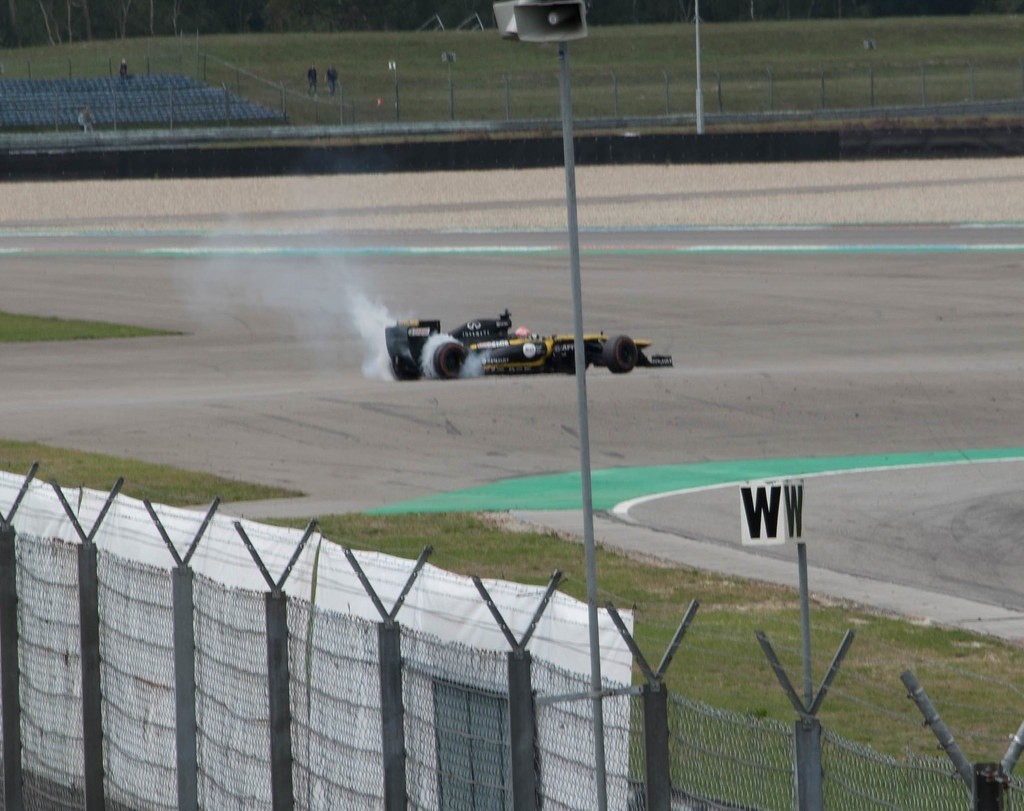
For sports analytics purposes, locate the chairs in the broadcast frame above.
[0,72,289,128]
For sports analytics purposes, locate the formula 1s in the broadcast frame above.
[385,307,672,379]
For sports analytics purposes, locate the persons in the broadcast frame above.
[120,59,127,84]
[325,63,337,96]
[307,64,318,98]
[82,105,94,133]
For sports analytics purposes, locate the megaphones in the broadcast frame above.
[493,0,518,40]
[514,0,588,42]
